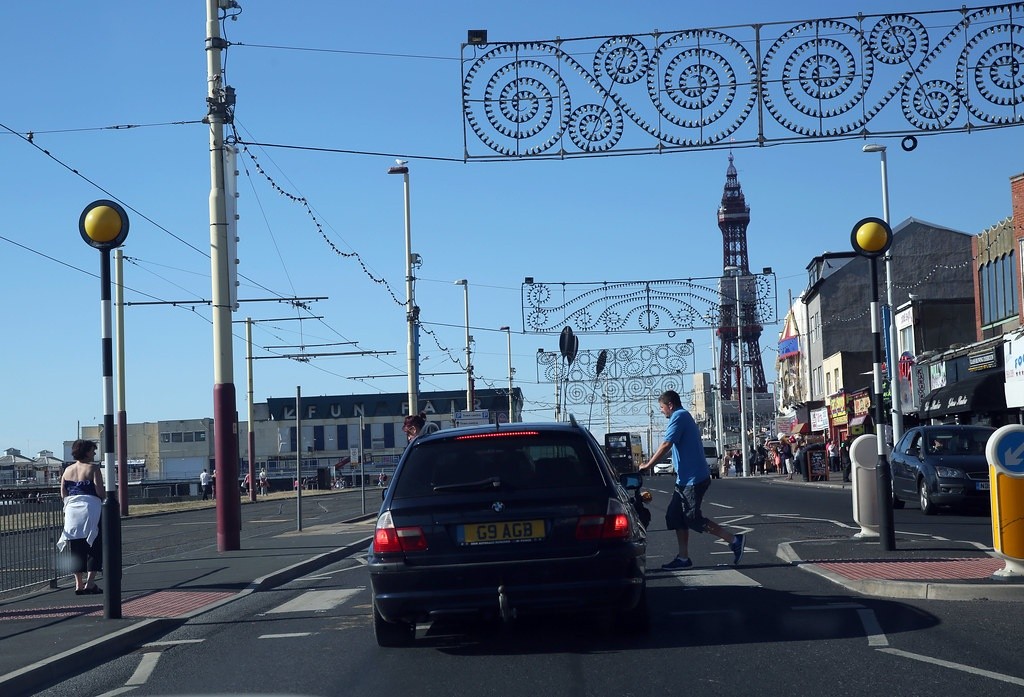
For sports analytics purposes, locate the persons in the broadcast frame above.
[244,473,249,497]
[200,469,216,500]
[259,467,268,496]
[840,435,852,482]
[331,478,347,489]
[826,439,840,472]
[639,390,746,569]
[402,411,426,442]
[378,473,387,487]
[724,439,793,480]
[57,439,105,595]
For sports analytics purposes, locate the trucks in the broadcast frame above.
[702,440,719,479]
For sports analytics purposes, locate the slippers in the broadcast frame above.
[75,590,83,595]
[83,584,103,595]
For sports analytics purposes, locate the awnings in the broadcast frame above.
[918,374,1005,419]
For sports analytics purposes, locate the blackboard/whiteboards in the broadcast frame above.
[808,451,828,475]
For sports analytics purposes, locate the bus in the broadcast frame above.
[604,431,643,473]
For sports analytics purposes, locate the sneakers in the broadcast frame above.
[662,554,693,570]
[728,534,746,564]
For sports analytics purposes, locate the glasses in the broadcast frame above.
[405,428,413,436]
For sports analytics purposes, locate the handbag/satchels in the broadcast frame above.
[260,479,264,487]
[241,480,247,488]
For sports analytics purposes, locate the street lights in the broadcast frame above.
[601,372,611,433]
[548,353,561,422]
[702,315,722,467]
[850,217,897,552]
[724,265,750,477]
[644,386,653,457]
[78,198,130,620]
[767,381,777,417]
[386,166,421,417]
[499,326,514,423]
[454,279,475,412]
[861,143,904,447]
[709,346,721,400]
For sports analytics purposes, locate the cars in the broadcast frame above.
[654,458,674,476]
[887,424,999,515]
[366,412,649,648]
